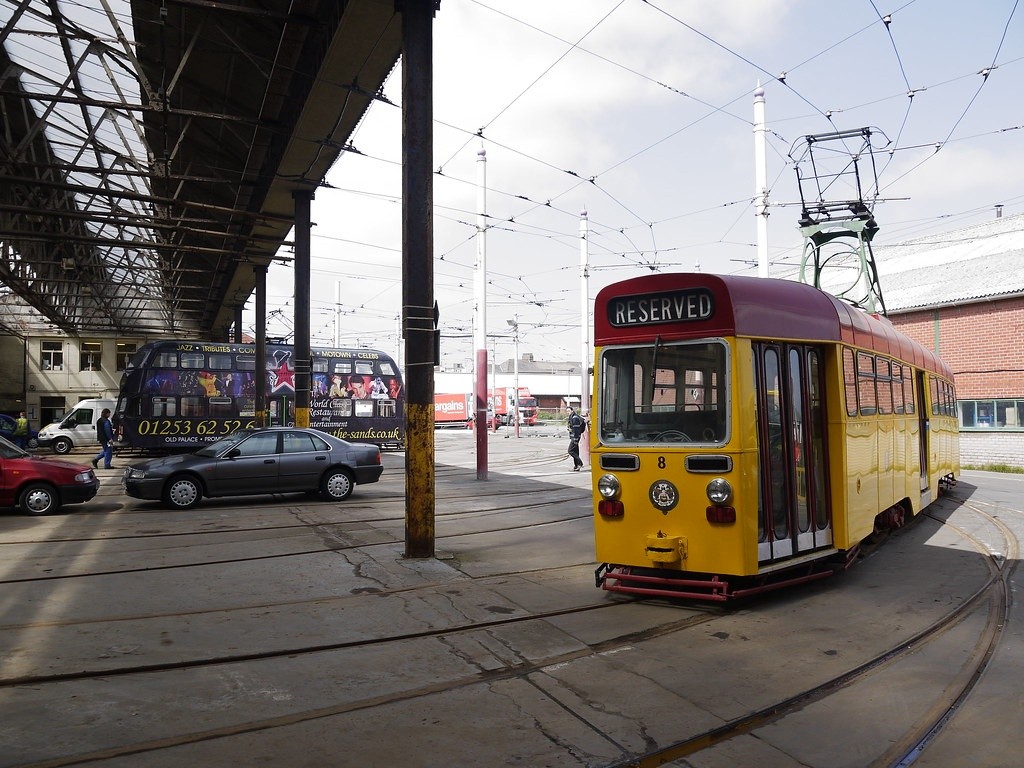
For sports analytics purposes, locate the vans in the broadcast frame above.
[37,397,186,455]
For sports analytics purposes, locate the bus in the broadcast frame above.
[586,125,961,604]
[110,339,407,451]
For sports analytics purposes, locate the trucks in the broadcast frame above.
[434,392,474,429]
[486,387,539,426]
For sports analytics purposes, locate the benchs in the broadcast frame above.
[635,402,703,435]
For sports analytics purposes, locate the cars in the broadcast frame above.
[466,411,502,431]
[120,425,384,510]
[0,434,101,516]
[0,414,40,449]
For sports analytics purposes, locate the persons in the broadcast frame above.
[14,411,31,450]
[331,375,402,400]
[93,409,115,469]
[199,372,234,397]
[566,407,583,471]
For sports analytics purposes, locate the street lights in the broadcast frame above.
[506,313,520,438]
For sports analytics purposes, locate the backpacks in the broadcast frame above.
[573,414,586,433]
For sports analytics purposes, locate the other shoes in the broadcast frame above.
[574,463,583,471]
[92,460,98,469]
[105,466,114,469]
